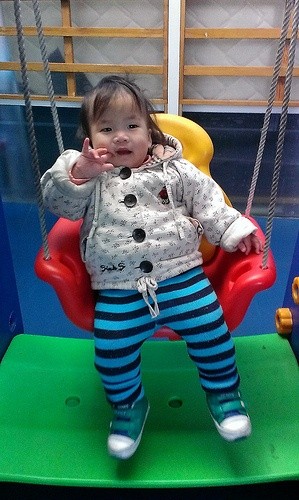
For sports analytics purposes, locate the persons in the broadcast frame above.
[39,74,264,461]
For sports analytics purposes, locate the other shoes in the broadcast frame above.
[204,390,252,443]
[105,396,150,459]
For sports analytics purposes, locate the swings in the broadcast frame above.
[14,0,299,341]
